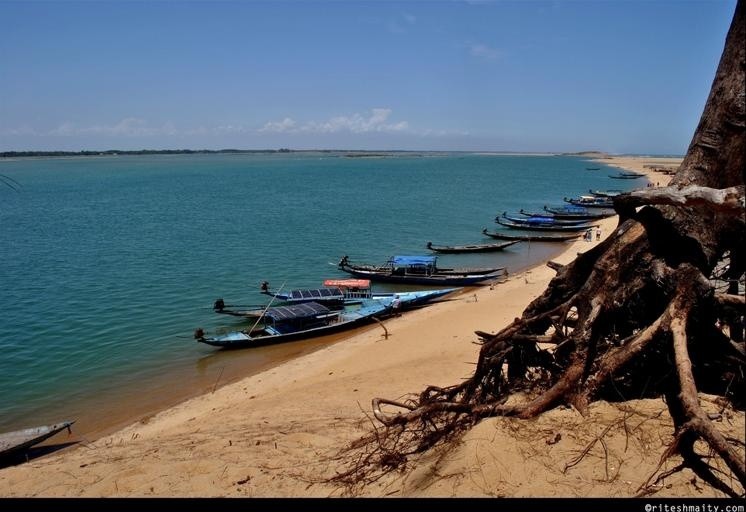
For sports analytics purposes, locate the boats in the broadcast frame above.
[195,189,624,350]
[607,173,645,179]
[0,420,76,455]
